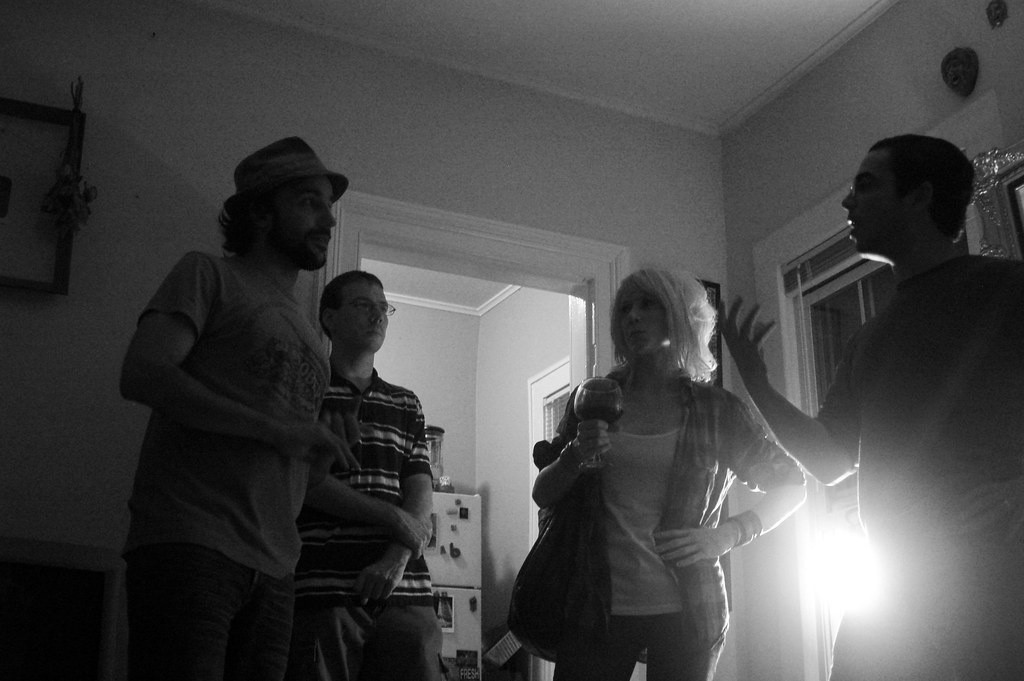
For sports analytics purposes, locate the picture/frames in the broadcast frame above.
[971,139,1024,262]
[1,93,85,294]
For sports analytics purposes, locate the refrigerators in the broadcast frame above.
[419,492,483,681]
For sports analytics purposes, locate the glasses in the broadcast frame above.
[336,299,396,318]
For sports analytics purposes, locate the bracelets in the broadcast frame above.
[561,438,607,477]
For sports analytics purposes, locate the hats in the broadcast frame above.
[223,136,349,215]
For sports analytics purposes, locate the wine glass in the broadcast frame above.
[573,377,625,474]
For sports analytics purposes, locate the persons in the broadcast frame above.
[716,134,1024,681]
[528,263,808,680]
[118,136,364,681]
[294,269,445,681]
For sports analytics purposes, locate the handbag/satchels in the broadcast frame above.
[507,474,611,662]
